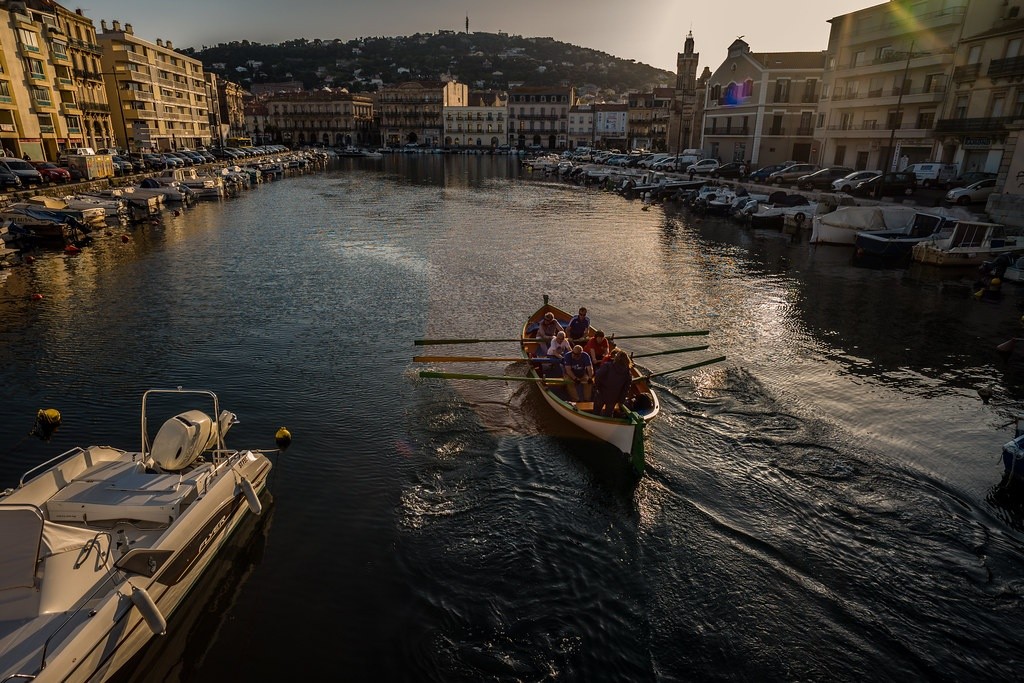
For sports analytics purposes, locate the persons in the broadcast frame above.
[5,148,14,158]
[657,143,664,152]
[563,345,595,402]
[566,308,590,348]
[593,351,633,418]
[536,312,564,356]
[22,152,31,160]
[547,331,572,368]
[56,150,61,163]
[583,331,619,368]
[718,156,723,166]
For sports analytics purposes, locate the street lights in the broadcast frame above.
[873,51,930,201]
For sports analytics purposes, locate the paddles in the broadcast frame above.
[412,336,551,347]
[606,330,709,339]
[420,371,577,383]
[413,355,563,362]
[629,345,711,358]
[649,356,727,378]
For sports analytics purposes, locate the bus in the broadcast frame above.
[224,137,252,148]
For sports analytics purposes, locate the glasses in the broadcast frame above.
[544,318,551,322]
[579,313,586,316]
[571,351,580,356]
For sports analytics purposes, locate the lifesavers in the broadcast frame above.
[794,212,806,223]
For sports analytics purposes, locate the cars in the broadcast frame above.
[528,144,541,149]
[208,144,289,160]
[707,163,917,198]
[0,157,70,191]
[896,163,998,205]
[562,146,720,174]
[58,147,216,172]
[497,144,509,150]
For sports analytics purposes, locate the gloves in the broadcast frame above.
[574,378,581,385]
[587,377,593,384]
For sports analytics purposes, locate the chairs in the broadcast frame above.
[0,501,44,591]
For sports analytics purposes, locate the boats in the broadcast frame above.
[216,149,327,187]
[809,206,920,246]
[0,168,223,237]
[912,221,1024,266]
[979,252,1024,283]
[0,390,273,683]
[854,212,961,257]
[520,295,659,473]
[528,157,620,182]
[615,170,818,226]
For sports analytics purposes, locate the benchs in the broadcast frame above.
[572,401,620,412]
